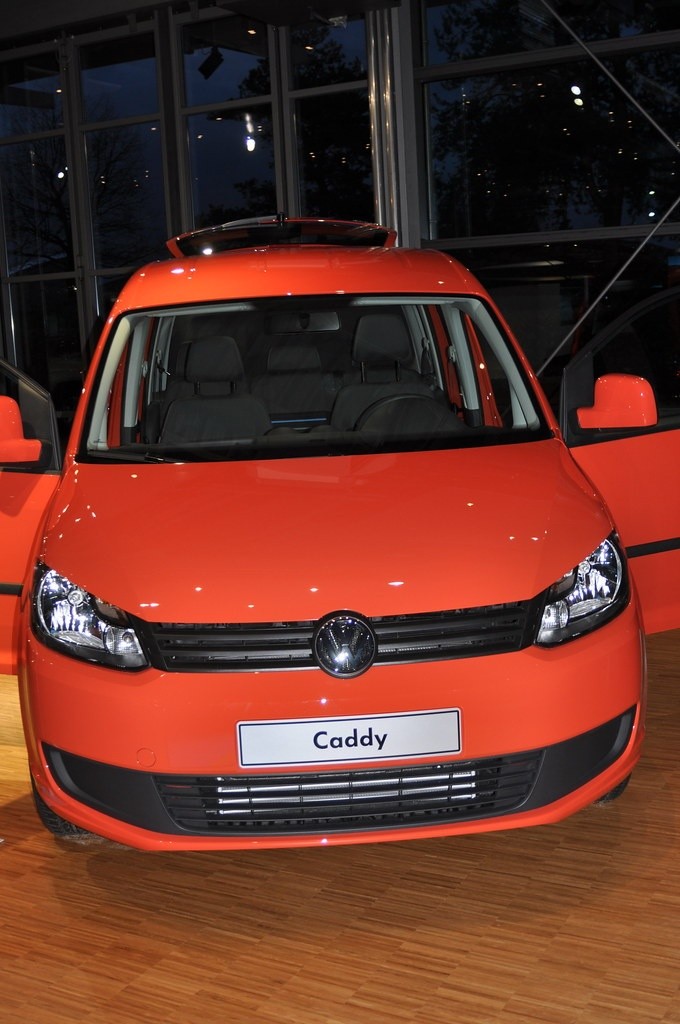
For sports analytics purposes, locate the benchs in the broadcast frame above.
[142,365,460,441]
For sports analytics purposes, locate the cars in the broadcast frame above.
[0,212,678,852]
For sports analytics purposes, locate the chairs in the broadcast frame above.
[156,332,276,445]
[330,309,444,437]
